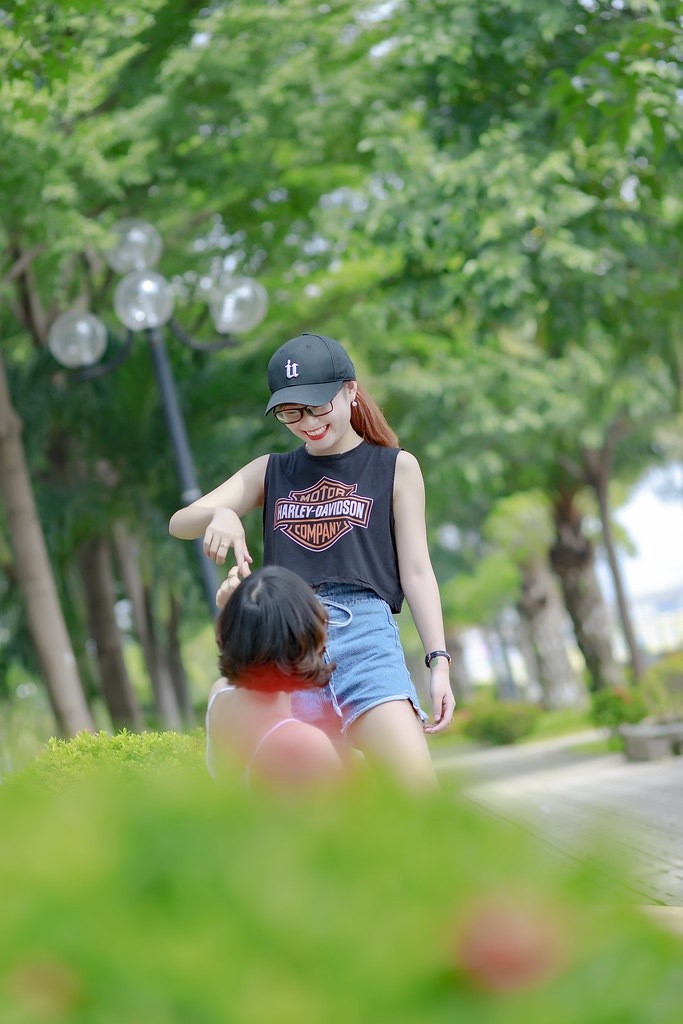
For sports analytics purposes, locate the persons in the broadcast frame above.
[169,333,456,793]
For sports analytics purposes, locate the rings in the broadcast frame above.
[228,573,236,578]
[221,543,229,549]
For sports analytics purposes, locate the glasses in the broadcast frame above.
[272,381,347,424]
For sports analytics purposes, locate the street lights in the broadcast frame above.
[45,214,268,627]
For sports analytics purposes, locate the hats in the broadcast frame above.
[264,332,356,416]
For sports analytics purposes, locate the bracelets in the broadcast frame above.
[425,650,451,668]
